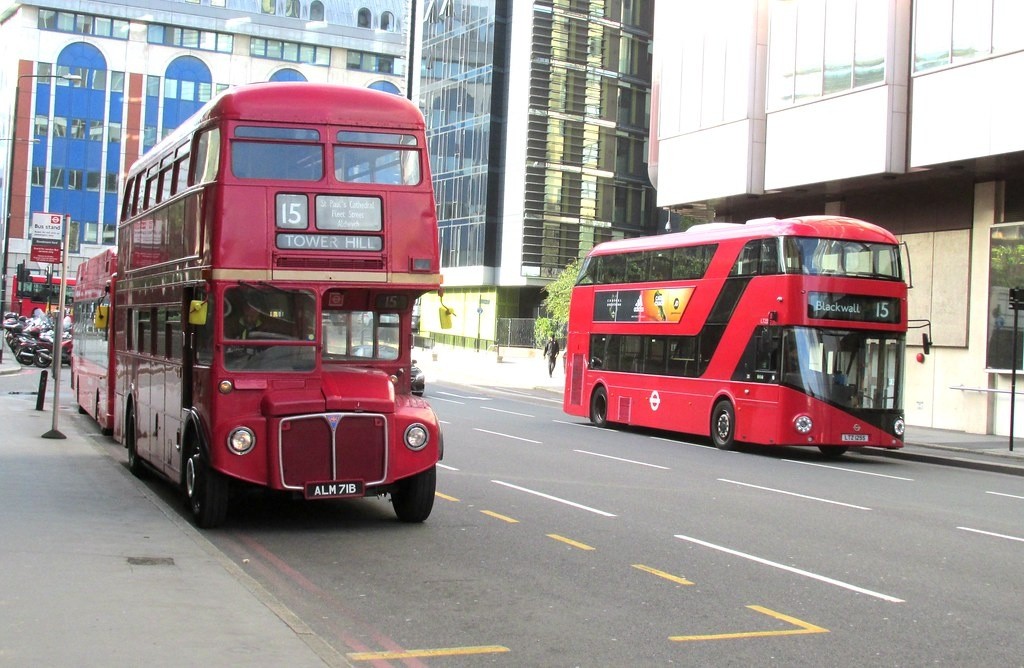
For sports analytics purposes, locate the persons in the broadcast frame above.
[992,308,1004,327]
[544,335,559,378]
[223,294,272,363]
[786,346,799,372]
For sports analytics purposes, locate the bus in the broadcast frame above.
[114,80,454,524]
[987,326,1023,369]
[71,247,117,436]
[11,275,77,318]
[382,297,420,331]
[563,214,932,457]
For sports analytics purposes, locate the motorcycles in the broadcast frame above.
[2,309,72,368]
[73,316,105,337]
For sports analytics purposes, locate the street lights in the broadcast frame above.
[1,74,83,312]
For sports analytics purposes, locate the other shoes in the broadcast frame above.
[550,375,552,378]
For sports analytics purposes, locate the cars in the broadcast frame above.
[322,314,330,326]
[358,311,374,328]
[348,344,426,397]
